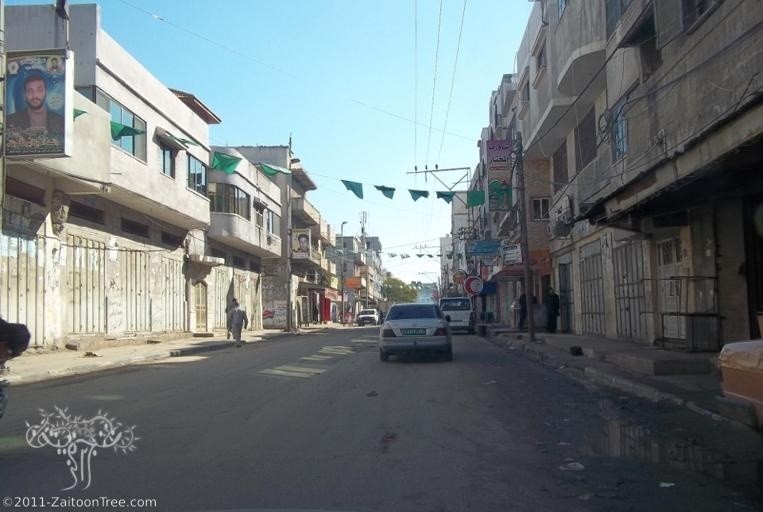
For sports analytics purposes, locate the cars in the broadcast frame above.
[356,308,379,327]
[379,302,454,362]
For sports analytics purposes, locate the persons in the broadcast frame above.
[292,234,310,257]
[312,303,318,324]
[48,57,62,73]
[544,288,559,331]
[226,299,248,347]
[379,311,384,324]
[519,294,527,329]
[0,320,30,417]
[6,75,64,138]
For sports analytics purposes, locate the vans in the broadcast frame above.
[438,297,476,334]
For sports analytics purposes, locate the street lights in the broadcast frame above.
[341,221,348,324]
